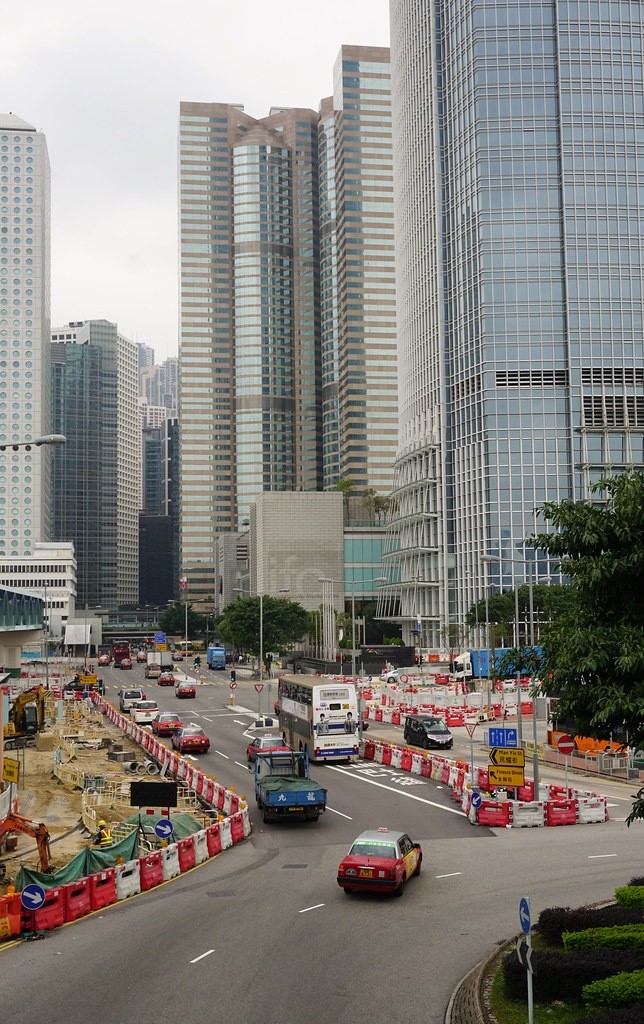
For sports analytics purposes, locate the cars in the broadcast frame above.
[171,725,210,753]
[100,655,110,661]
[246,734,292,761]
[139,689,146,699]
[172,653,183,661]
[274,701,280,716]
[151,712,184,736]
[98,657,109,666]
[144,663,161,679]
[121,658,132,669]
[157,671,175,685]
[174,681,196,699]
[358,714,369,730]
[336,826,423,899]
[137,652,147,662]
[380,670,398,684]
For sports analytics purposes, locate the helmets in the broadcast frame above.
[98,820,107,827]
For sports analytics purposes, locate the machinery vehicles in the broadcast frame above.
[0,813,56,884]
[4,684,54,752]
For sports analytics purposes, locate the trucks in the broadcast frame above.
[207,647,226,669]
[147,652,174,671]
[99,644,112,661]
[247,751,328,824]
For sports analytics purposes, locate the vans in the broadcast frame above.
[64,679,105,697]
[117,688,142,712]
[404,714,454,749]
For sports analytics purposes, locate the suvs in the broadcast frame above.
[129,701,159,724]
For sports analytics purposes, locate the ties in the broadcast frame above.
[321,721,324,725]
[349,720,351,725]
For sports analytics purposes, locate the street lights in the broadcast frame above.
[85,604,100,671]
[318,575,387,694]
[168,599,205,679]
[465,554,573,799]
[135,604,170,646]
[233,588,291,680]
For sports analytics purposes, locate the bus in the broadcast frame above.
[277,674,359,762]
[113,641,131,667]
[175,641,193,655]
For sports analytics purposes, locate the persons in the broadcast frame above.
[343,712,357,734]
[264,654,273,676]
[316,713,329,735]
[430,723,437,729]
[237,653,245,666]
[246,652,251,663]
[194,656,201,666]
[93,820,113,849]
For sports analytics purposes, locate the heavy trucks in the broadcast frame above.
[449,645,543,684]
